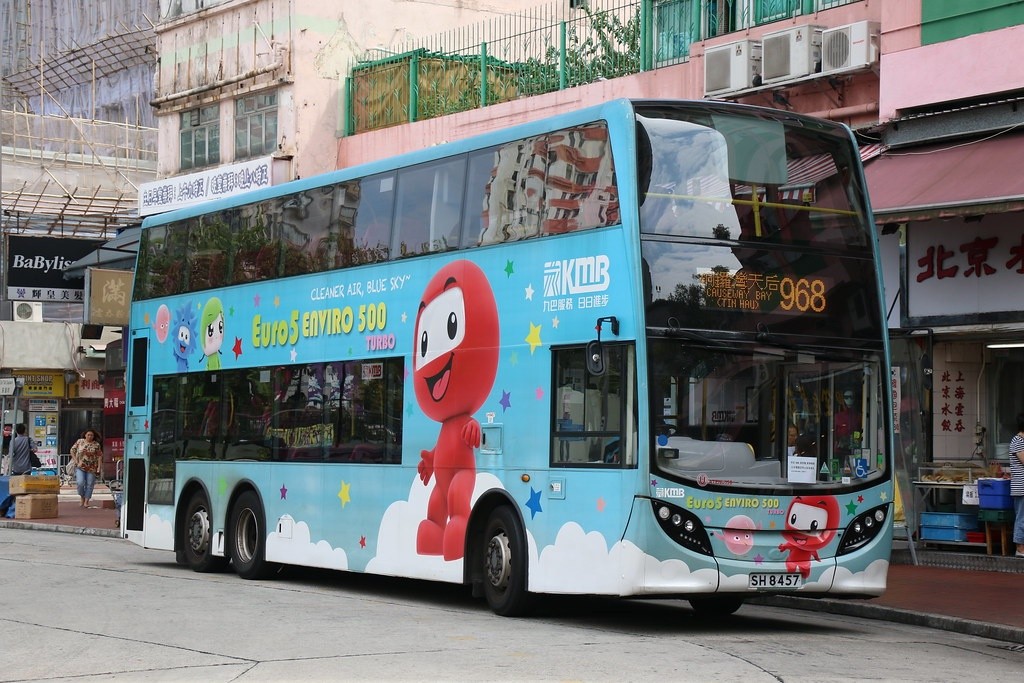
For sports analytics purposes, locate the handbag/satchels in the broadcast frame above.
[28,436,42,469]
[65,458,77,477]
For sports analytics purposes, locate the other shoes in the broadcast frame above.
[84,503,90,508]
[79,502,84,507]
[1015,550,1024,558]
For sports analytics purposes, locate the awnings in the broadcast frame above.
[653,142,892,208]
[0,321,127,370]
[62,228,143,279]
[810,130,1024,228]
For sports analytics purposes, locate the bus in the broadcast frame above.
[120,97,897,617]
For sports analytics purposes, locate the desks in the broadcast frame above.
[913,484,1010,556]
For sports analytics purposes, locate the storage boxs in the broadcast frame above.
[978,477,1014,521]
[918,512,985,542]
[15,493,58,519]
[9,475,60,495]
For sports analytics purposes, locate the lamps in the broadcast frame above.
[881,223,900,235]
[962,214,984,222]
[65,371,78,384]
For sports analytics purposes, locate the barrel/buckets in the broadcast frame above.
[995,443,1010,460]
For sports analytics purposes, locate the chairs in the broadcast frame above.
[201,390,401,463]
[586,388,622,457]
[164,215,426,291]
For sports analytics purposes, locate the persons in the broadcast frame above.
[796,433,830,480]
[200,392,221,457]
[4,423,38,475]
[181,425,192,457]
[835,390,862,466]
[788,424,798,457]
[1008,413,1024,557]
[70,429,102,508]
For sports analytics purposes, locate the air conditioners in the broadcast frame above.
[13,300,43,322]
[821,20,881,73]
[761,23,827,84]
[703,38,762,96]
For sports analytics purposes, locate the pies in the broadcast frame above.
[921,473,969,482]
